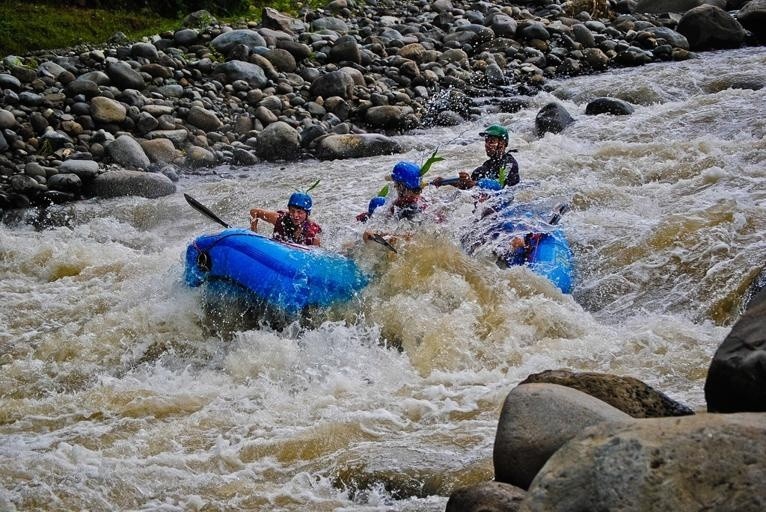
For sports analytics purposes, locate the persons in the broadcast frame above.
[433,125,521,221]
[249,192,321,247]
[357,160,427,244]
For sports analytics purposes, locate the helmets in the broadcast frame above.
[477,126,509,147]
[287,192,313,212]
[391,160,423,190]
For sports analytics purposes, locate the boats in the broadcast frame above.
[361,167,538,217]
[178,212,580,342]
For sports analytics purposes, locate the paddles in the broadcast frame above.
[184,194,231,228]
